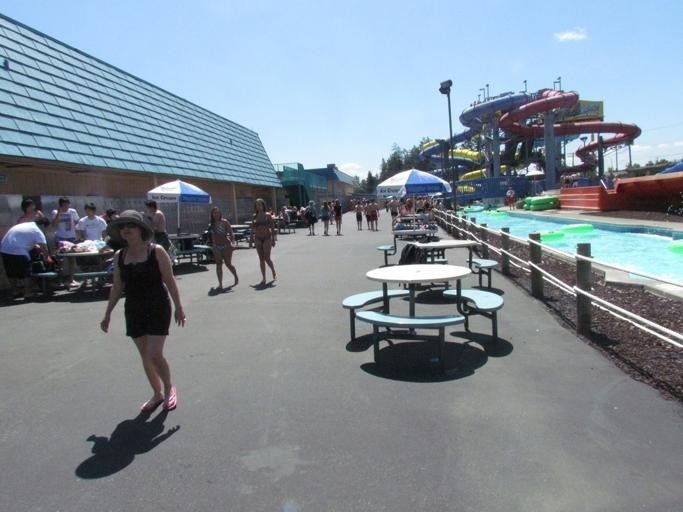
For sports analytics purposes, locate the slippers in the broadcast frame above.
[163,386,177,410]
[140,396,164,412]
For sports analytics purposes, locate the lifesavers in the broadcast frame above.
[669,240,682,254]
[534,230,565,241]
[562,224,594,234]
[524,196,560,211]
[466,207,473,210]
[483,210,508,217]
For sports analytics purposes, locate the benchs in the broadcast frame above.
[341,213,505,378]
[28,220,306,302]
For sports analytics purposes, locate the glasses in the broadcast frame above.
[118,222,136,228]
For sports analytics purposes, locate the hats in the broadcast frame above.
[106,210,152,241]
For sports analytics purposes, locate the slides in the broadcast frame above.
[500,90,642,174]
[420,91,549,195]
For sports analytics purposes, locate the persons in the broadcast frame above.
[506,186,516,211]
[664,191,683,216]
[100,209,186,412]
[349,196,443,232]
[560,172,578,189]
[249,198,278,285]
[0,196,120,301]
[268,199,342,235]
[145,199,170,250]
[207,207,238,291]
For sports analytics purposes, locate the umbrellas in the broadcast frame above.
[146,177,212,236]
[376,167,452,230]
